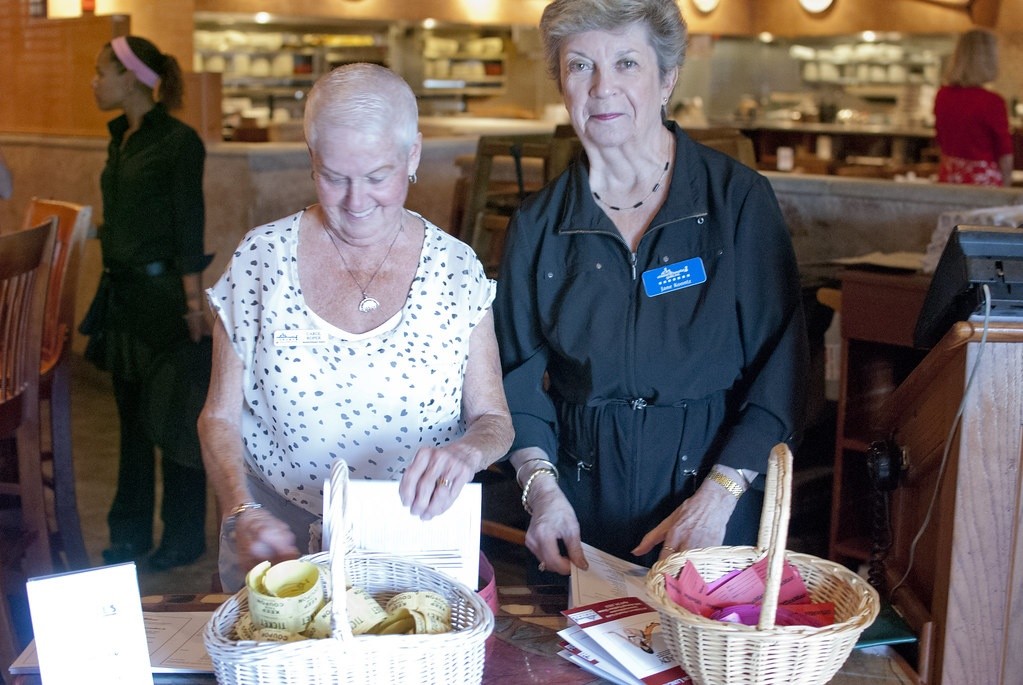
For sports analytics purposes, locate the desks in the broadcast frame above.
[139,585,921,685]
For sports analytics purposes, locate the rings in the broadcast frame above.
[538,560,546,572]
[660,546,677,554]
[436,477,452,489]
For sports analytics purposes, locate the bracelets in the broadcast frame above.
[516,458,560,516]
[737,469,753,488]
[704,469,744,502]
[221,501,263,544]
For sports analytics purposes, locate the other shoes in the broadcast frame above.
[141,544,206,571]
[101,542,155,564]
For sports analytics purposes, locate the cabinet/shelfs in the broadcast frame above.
[403,18,511,97]
[828,265,932,565]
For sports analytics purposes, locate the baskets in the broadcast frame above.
[641,443,881,685]
[202,458,495,685]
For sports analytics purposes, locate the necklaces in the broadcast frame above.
[586,129,672,211]
[323,208,403,312]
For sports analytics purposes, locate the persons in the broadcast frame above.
[493,0,814,596]
[931,28,1014,188]
[76,34,215,573]
[196,64,518,596]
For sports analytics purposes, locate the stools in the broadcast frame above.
[0,194,95,685]
